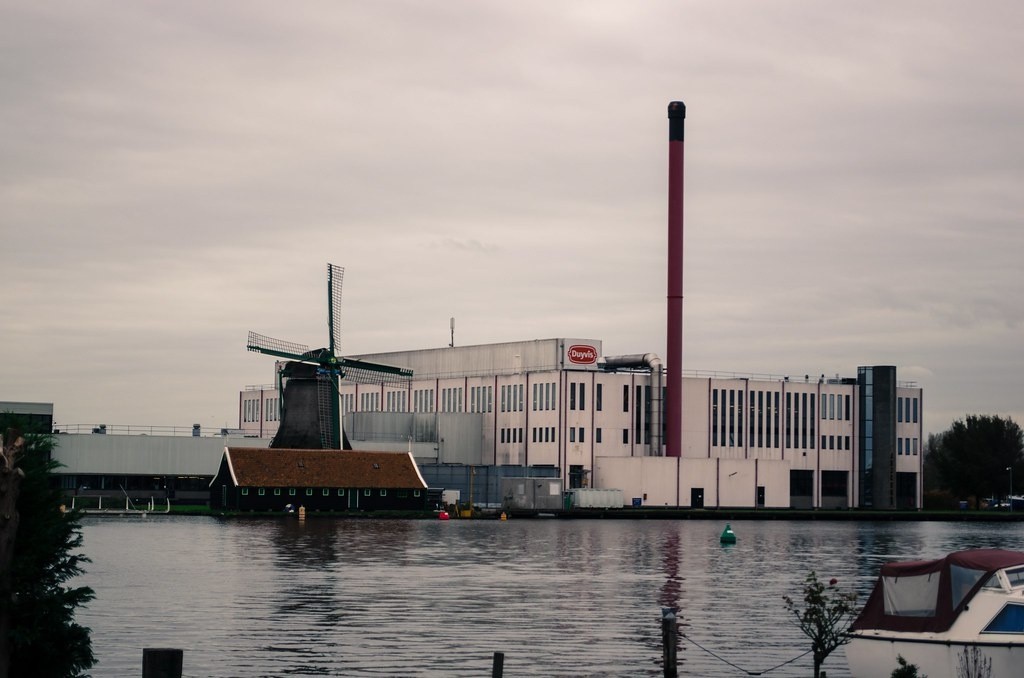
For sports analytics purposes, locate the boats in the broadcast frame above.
[835,545,1023,677]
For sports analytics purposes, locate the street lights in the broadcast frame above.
[1005,466,1012,512]
